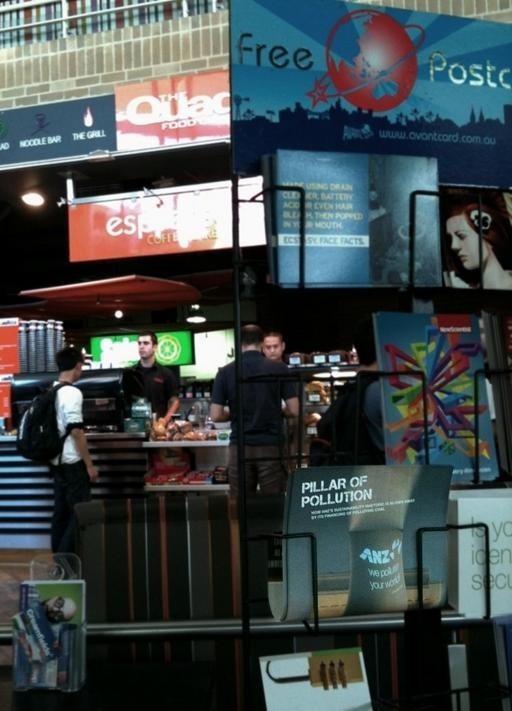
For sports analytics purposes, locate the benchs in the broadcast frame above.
[45,497,453,710]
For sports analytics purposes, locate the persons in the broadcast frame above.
[261,329,287,362]
[341,332,386,465]
[121,332,180,427]
[42,596,76,623]
[309,383,353,466]
[210,325,301,620]
[441,197,510,290]
[50,346,99,577]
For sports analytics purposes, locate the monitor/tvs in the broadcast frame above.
[9,370,59,425]
[65,369,131,429]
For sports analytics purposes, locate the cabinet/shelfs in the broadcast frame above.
[145,422,234,493]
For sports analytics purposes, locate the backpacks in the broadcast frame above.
[16,382,73,462]
